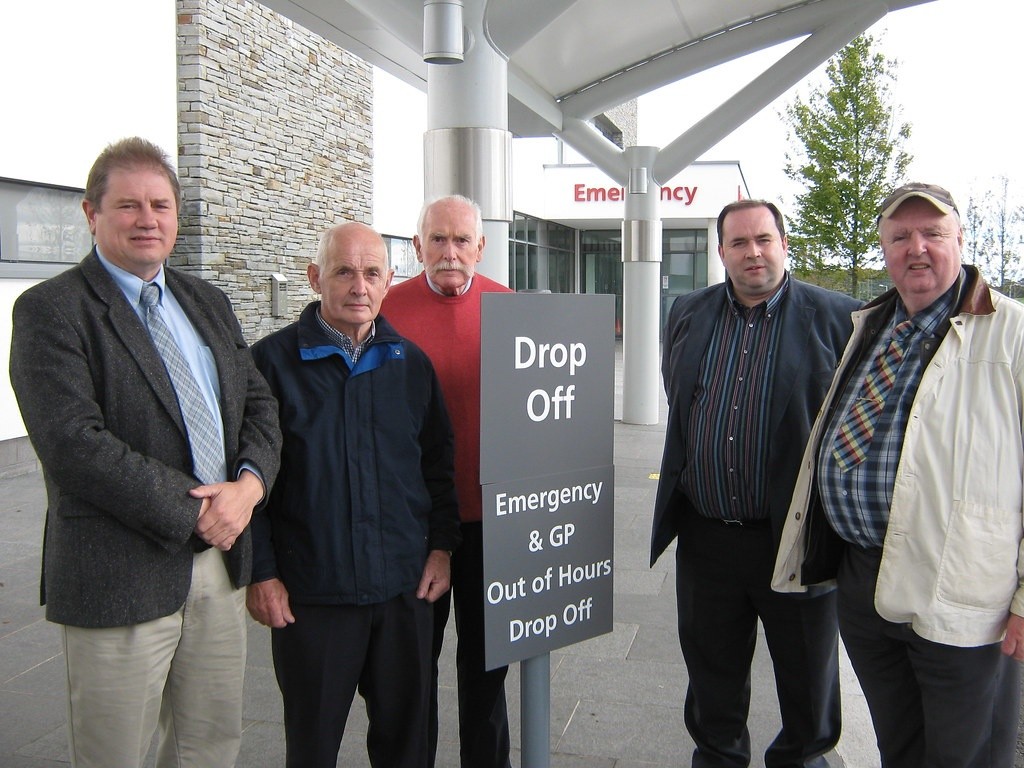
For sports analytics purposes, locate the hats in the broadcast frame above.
[877,182,960,229]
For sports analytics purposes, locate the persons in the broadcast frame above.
[376,194,521,768]
[644,200,870,768]
[8,138,284,768]
[243,222,460,768]
[769,180,1023,768]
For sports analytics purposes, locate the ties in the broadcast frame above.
[141,281,225,485]
[831,320,916,473]
